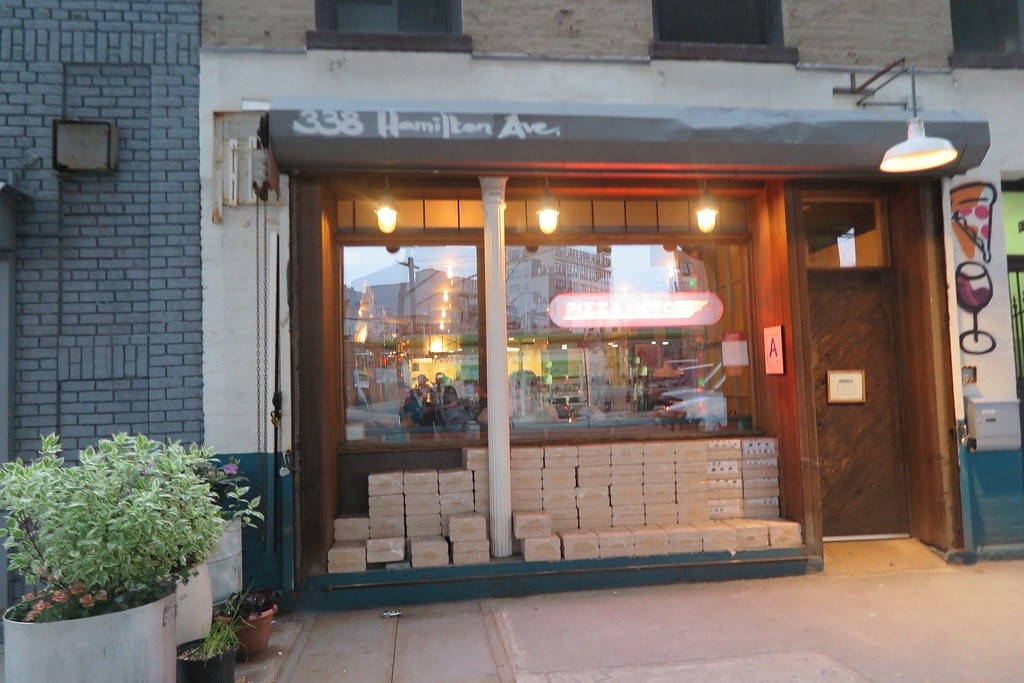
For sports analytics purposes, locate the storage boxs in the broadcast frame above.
[511,437,804,564]
[326,446,491,574]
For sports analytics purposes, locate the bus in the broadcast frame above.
[400,334,689,410]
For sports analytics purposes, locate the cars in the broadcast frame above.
[549,395,593,418]
[652,362,726,432]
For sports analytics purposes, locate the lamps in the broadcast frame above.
[852,62,958,174]
[693,177,721,234]
[372,176,400,235]
[535,177,561,235]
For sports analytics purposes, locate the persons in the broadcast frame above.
[626,373,687,414]
[478,369,560,424]
[402,373,485,438]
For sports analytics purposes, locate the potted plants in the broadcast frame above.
[0,428,280,683]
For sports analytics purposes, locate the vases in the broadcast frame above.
[205,516,244,606]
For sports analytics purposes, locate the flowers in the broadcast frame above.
[141,454,265,530]
